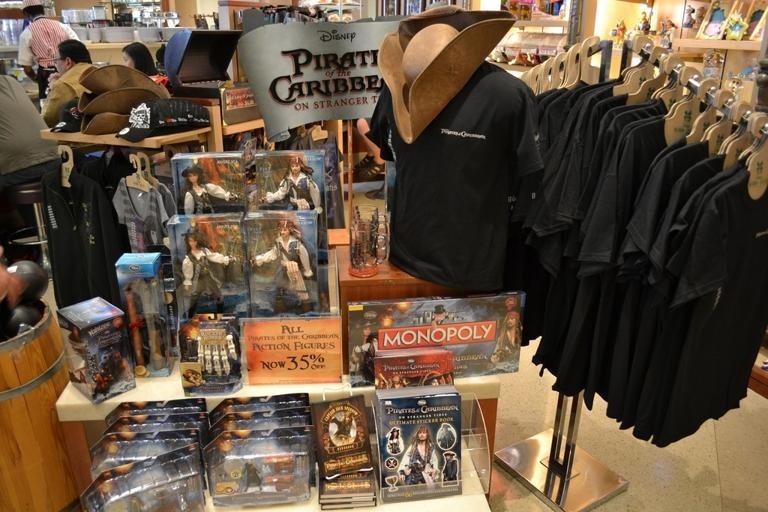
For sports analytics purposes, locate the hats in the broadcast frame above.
[79,65,170,135]
[378,4,516,145]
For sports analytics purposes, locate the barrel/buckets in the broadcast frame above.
[0,295,92,511]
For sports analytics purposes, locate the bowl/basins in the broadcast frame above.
[88,28,100,43]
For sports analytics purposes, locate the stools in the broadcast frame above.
[6,182,53,280]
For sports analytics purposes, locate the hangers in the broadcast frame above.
[125,143,189,195]
[521,36,600,97]
[613,36,767,200]
[55,144,77,190]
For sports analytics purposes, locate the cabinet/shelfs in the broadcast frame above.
[52,358,499,511]
[482,18,567,72]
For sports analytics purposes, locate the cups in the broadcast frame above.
[348,221,388,278]
[0,19,25,46]
[61,10,95,23]
[352,205,388,235]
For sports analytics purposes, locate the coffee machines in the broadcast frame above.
[114,8,140,28]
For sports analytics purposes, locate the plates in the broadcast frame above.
[100,27,183,43]
[73,28,87,41]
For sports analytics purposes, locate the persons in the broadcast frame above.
[350,115,386,181]
[248,220,314,312]
[41,39,101,129]
[386,421,461,492]
[0,73,64,192]
[181,164,239,215]
[179,226,237,312]
[362,5,547,295]
[16,0,82,111]
[243,462,264,493]
[121,40,168,90]
[491,311,517,373]
[258,158,322,217]
